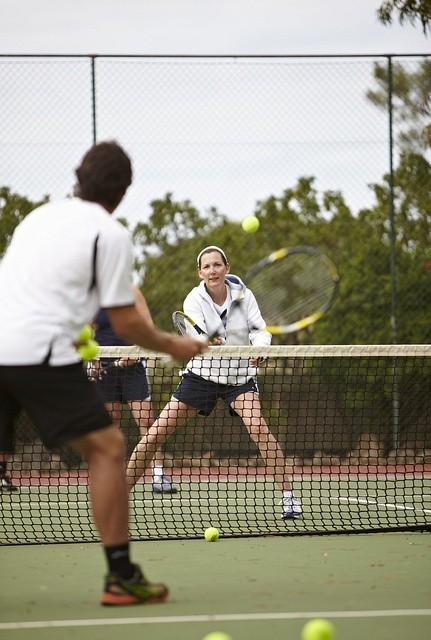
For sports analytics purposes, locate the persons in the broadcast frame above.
[0,141,207,605]
[88,282,178,493]
[125,246,304,519]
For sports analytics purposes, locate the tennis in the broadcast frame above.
[241,215,260,234]
[204,526,220,542]
[75,323,96,345]
[302,618,337,639]
[78,338,100,360]
[202,631,232,640]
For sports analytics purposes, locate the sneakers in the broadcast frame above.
[278,497,303,519]
[101,565,167,606]
[152,476,177,493]
[0,476,17,492]
